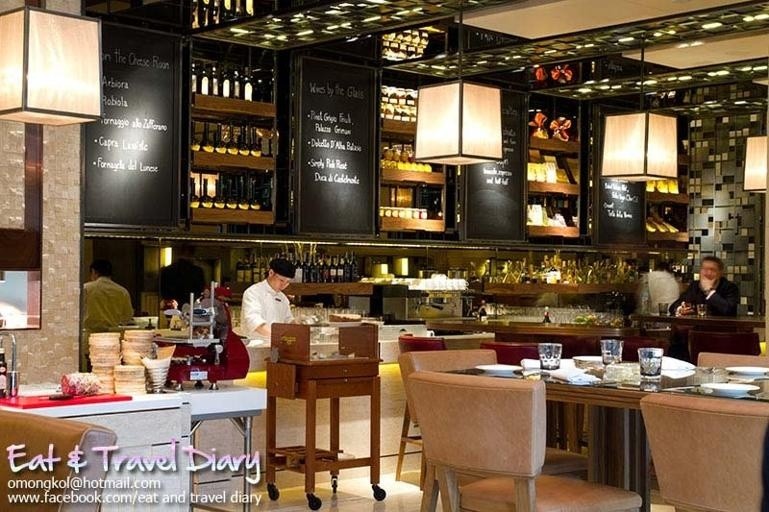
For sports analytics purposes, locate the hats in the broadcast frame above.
[269,258,295,279]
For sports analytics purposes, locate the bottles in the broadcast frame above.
[639,272,651,315]
[0,348,7,396]
[532,195,573,226]
[192,0,255,31]
[189,58,434,285]
[467,254,632,284]
[479,300,487,322]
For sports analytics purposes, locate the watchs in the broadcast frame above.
[703,287,715,295]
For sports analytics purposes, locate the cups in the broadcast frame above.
[636,346,665,383]
[599,338,625,365]
[230,305,366,322]
[537,343,563,371]
[697,304,707,317]
[658,302,668,315]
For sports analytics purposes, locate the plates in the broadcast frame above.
[700,383,760,396]
[474,364,523,375]
[725,367,769,376]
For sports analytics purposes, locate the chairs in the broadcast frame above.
[399,330,767,511]
[58,402,191,512]
[0,409,118,511]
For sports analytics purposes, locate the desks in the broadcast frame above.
[628,309,765,330]
[0,385,267,511]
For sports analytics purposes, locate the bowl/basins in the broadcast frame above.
[573,356,601,370]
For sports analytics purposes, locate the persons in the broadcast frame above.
[80,260,134,372]
[240,259,300,339]
[160,245,203,318]
[668,257,739,361]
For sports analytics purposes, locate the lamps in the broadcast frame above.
[410,2,505,169]
[599,31,680,184]
[740,134,767,195]
[0,1,107,128]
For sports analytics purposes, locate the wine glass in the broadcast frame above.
[498,304,614,323]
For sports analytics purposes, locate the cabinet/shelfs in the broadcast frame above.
[644,113,690,243]
[187,38,279,226]
[525,96,580,240]
[266,322,386,511]
[378,72,446,233]
